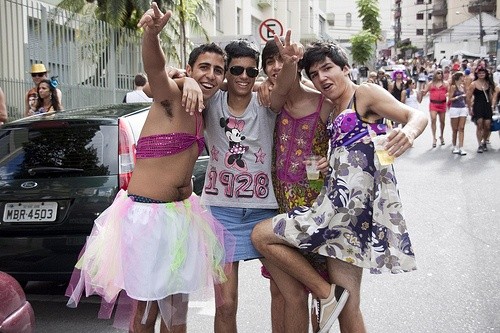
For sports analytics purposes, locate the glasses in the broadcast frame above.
[437,72,443,75]
[31,72,46,77]
[228,66,259,78]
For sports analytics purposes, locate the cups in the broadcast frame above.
[303,155,323,181]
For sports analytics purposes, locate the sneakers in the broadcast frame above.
[312,282,351,333]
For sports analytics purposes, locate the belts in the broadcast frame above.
[430,99,446,103]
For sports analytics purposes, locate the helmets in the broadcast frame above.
[497,65,500,71]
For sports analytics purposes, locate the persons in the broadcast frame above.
[67,2,430,333]
[122,74,149,103]
[349,55,500,156]
[24,64,65,116]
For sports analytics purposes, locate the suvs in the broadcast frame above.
[0,103,212,292]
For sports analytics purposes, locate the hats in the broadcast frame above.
[30,64,47,74]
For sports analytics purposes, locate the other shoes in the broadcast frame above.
[440,136,445,145]
[483,143,488,150]
[433,139,437,146]
[459,147,466,155]
[452,146,460,154]
[477,146,483,153]
